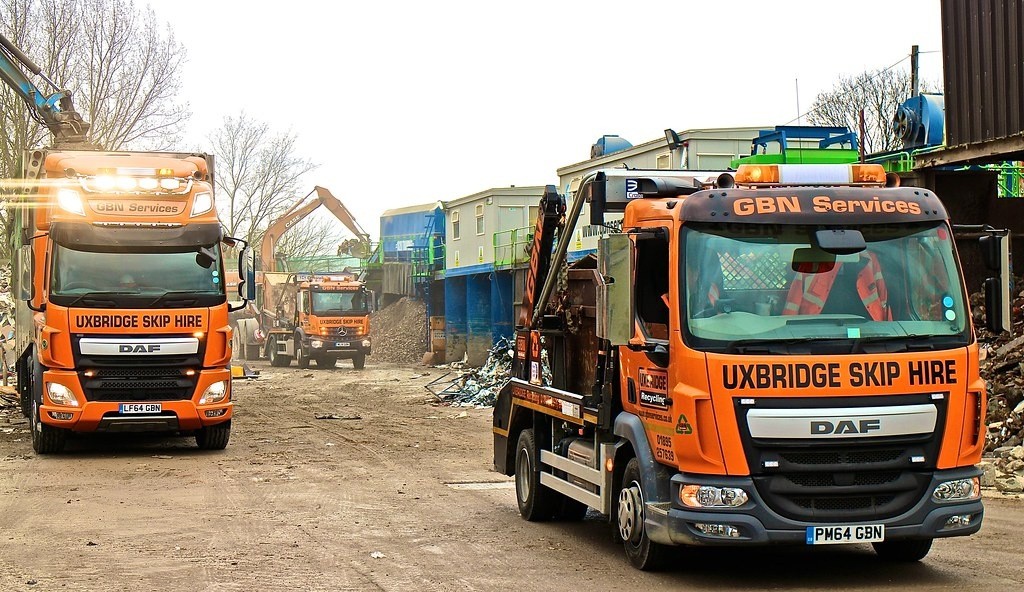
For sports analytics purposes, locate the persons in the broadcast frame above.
[683,246,724,319]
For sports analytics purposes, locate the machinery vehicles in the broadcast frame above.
[223,186,371,361]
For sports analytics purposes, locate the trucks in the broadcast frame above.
[263,272,372,369]
[11,151,254,455]
[491,165,984,573]
